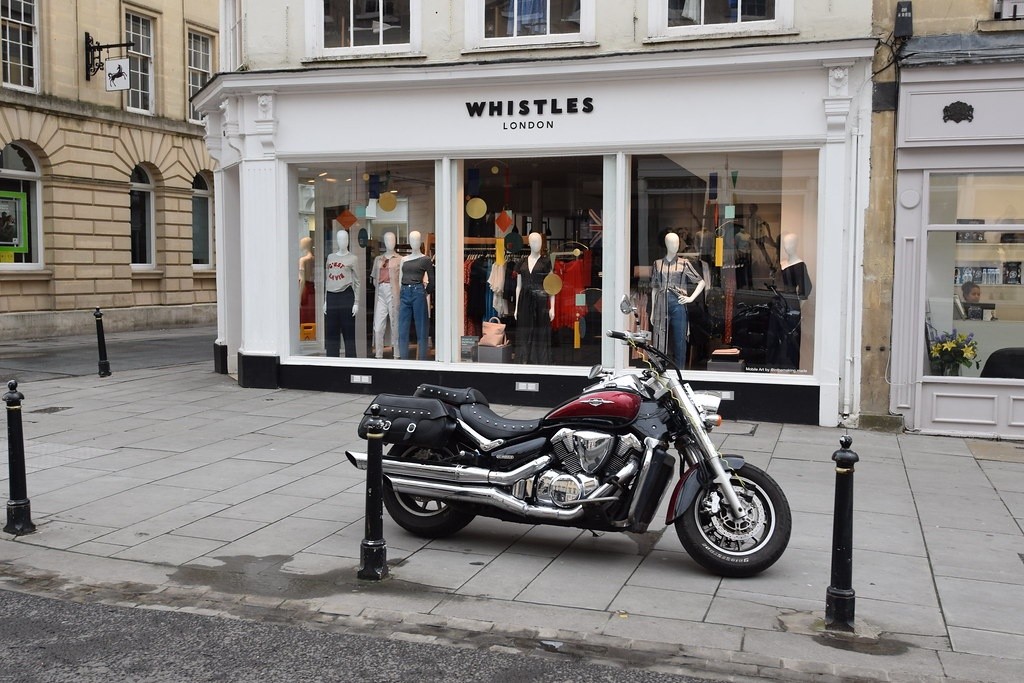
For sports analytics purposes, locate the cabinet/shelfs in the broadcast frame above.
[953,219,1024,321]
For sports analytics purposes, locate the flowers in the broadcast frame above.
[930,328,982,370]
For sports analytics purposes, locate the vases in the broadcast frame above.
[941,363,962,376]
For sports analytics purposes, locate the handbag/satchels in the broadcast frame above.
[477,317,506,346]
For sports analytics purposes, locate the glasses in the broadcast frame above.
[383,258,389,267]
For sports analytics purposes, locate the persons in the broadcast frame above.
[297,237,315,329]
[746,204,779,279]
[322,229,361,358]
[370,229,434,361]
[765,233,813,371]
[962,281,981,302]
[513,232,555,365]
[649,232,706,370]
[694,224,758,292]
[0,212,13,232]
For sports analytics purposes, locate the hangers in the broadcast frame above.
[430,251,529,262]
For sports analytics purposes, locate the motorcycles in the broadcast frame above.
[343,294,793,579]
